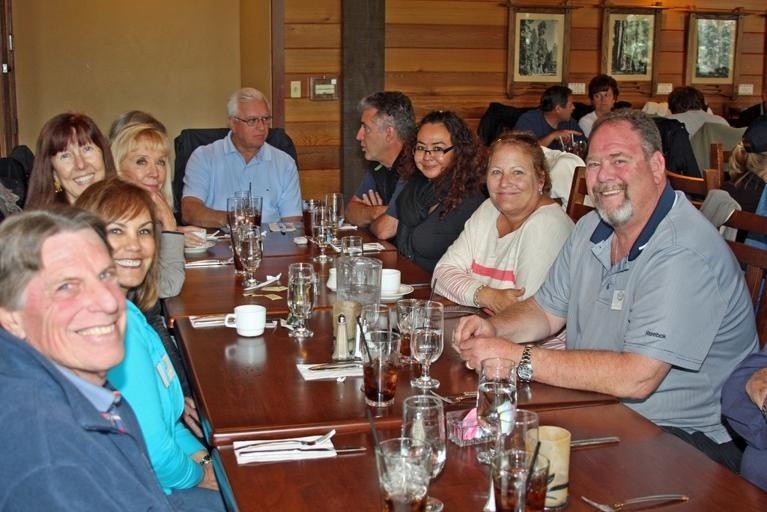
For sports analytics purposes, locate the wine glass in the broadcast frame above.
[402,395,448,511]
[224,182,445,388]
[476,356,548,511]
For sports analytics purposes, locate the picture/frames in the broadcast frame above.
[600,4,662,96]
[682,7,743,100]
[505,4,570,101]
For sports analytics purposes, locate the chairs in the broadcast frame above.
[1,128,299,224]
[485,101,766,351]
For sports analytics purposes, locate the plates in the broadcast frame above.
[184,242,216,254]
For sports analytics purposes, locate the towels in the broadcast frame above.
[296,358,364,383]
[330,238,384,255]
[229,428,338,466]
[185,308,232,329]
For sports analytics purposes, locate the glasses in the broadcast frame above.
[411,145,453,158]
[235,114,273,127]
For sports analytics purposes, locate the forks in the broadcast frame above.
[234,429,336,450]
[579,493,689,511]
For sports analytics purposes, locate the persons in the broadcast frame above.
[728,75,767,318]
[513,86,589,149]
[346,91,574,350]
[181,87,303,228]
[0,112,225,511]
[721,343,767,493]
[664,86,729,139]
[452,107,760,471]
[579,74,619,140]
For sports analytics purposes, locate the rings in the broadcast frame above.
[465,360,475,370]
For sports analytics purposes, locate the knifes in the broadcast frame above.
[239,447,367,453]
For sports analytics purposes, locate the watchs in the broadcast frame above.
[517,343,536,382]
[761,393,767,418]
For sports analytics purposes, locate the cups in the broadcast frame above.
[374,438,430,511]
[359,331,400,409]
[187,228,206,245]
[559,134,586,155]
[525,426,570,510]
